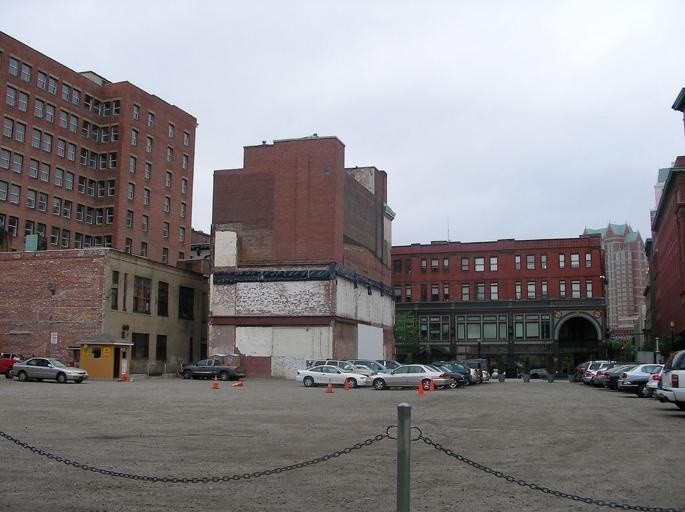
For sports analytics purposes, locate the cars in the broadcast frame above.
[13,356,89,384]
[572,350,685,416]
[295,355,493,393]
[529,368,549,377]
[0,351,21,380]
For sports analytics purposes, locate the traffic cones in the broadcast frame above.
[211,374,221,391]
[232,378,244,387]
[416,380,425,397]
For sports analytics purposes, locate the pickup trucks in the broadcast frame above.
[178,358,249,381]
